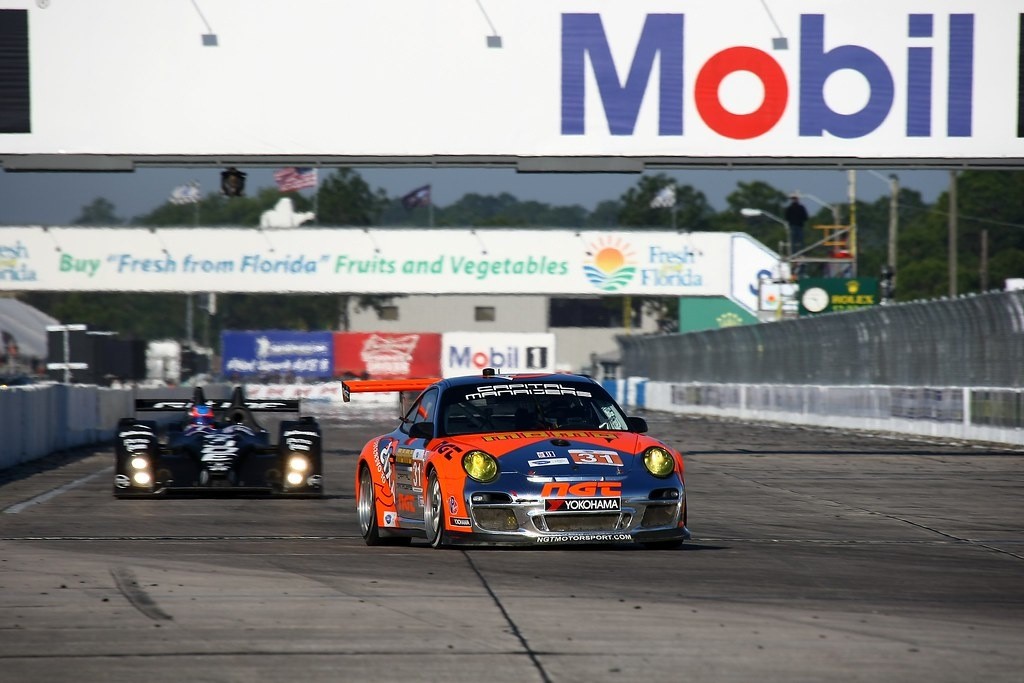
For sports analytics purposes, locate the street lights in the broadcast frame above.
[740,203,792,314]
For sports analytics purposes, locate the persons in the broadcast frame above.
[784,196,808,256]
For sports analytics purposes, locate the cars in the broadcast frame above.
[352,370,691,546]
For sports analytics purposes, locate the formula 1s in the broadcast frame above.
[109,384,325,502]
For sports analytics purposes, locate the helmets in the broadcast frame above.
[537,395,584,428]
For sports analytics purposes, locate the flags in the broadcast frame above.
[275,167,317,192]
[168,180,199,205]
[401,184,432,211]
[650,183,676,207]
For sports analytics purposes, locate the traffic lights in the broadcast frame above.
[221,170,246,197]
